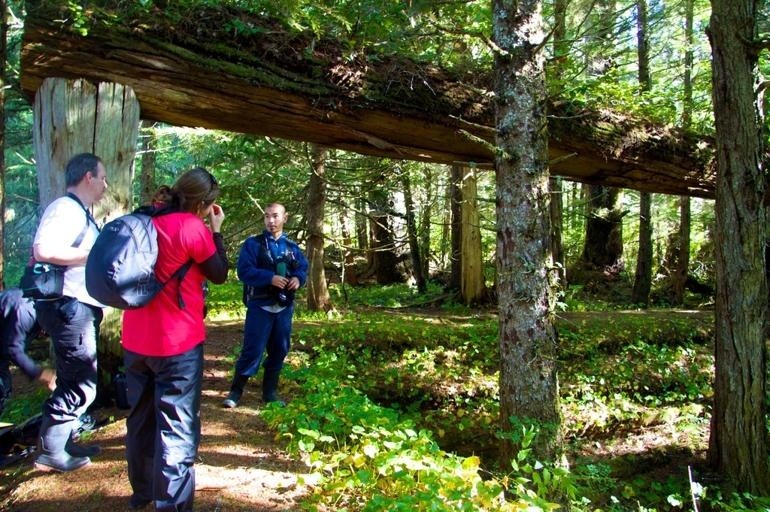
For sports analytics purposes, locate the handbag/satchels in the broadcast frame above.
[21,262,64,298]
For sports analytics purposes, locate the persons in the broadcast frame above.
[0,286,58,418]
[120,164,229,512]
[220,201,310,411]
[34,151,109,472]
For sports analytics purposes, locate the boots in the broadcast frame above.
[262,368,287,407]
[221,373,249,408]
[34,415,103,474]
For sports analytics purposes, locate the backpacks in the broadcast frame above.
[85,205,193,309]
